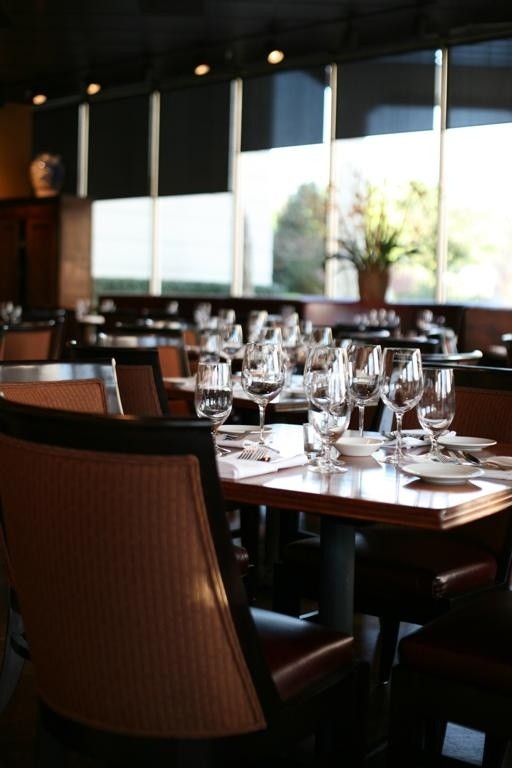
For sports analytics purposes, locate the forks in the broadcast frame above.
[236,449,269,467]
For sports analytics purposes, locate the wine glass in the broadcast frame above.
[195,362,233,458]
[416,366,461,465]
[218,324,243,386]
[241,343,285,448]
[346,344,383,435]
[380,347,425,464]
[306,346,353,475]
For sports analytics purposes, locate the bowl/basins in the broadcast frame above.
[331,436,384,456]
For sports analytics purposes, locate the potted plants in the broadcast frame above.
[321,201,427,305]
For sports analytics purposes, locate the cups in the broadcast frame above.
[302,424,323,453]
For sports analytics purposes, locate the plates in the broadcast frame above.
[401,463,484,485]
[437,435,498,452]
[385,428,458,448]
[215,423,273,438]
